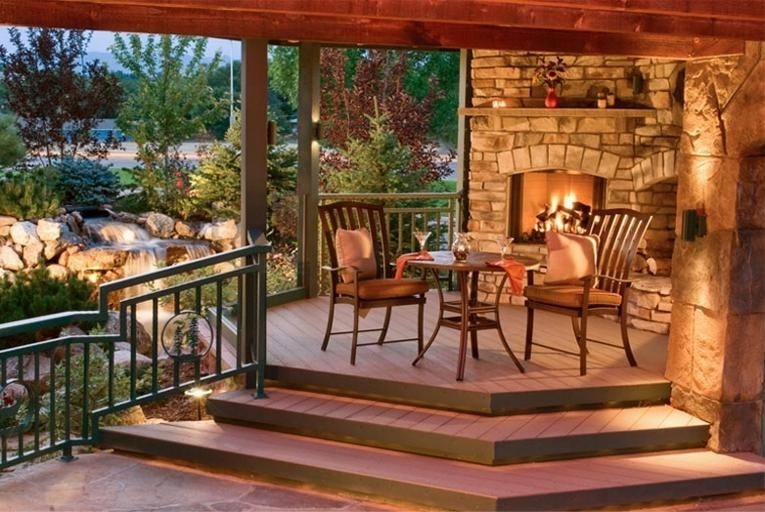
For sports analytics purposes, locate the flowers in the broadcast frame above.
[534,60,567,87]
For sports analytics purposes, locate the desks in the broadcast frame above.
[403,249,539,382]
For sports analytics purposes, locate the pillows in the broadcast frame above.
[336,227,377,283]
[544,230,600,289]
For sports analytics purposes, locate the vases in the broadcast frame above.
[544,88,559,108]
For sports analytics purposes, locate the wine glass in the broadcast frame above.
[412,230,432,255]
[494,235,516,261]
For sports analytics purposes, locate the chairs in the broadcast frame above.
[523,208,654,376]
[316,201,427,365]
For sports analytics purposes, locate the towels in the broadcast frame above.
[393,249,433,280]
[488,257,527,298]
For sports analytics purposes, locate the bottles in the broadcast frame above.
[451,232,472,262]
[597,91,615,108]
